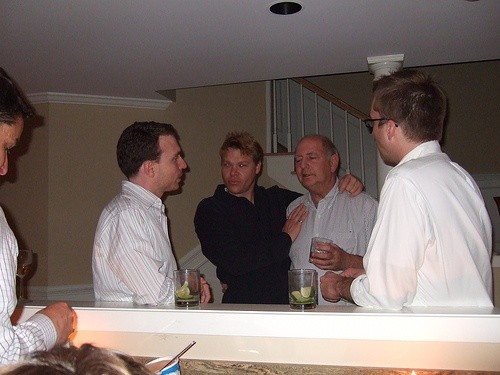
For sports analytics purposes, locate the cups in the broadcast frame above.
[309,236,333,264]
[173,269,202,307]
[144,355,181,375]
[288,269,319,310]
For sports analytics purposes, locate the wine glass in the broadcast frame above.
[14,249,35,304]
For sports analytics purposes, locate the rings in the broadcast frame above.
[298,219,302,224]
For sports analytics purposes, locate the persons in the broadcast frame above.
[0,66,76,364]
[1,343,143,375]
[193,131,364,305]
[91,121,212,305]
[319,67,497,309]
[284,134,380,305]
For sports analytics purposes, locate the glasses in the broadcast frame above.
[363,118,398,134]
